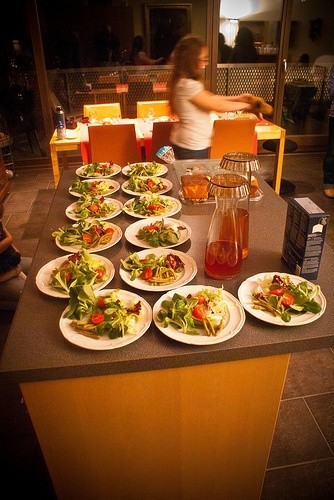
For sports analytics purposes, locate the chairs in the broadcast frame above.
[151,122,177,164]
[82,102,122,119]
[137,101,170,118]
[209,119,257,161]
[88,123,137,166]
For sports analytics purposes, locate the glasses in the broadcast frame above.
[198,58,209,62]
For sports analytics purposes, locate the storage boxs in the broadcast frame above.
[280,197,330,280]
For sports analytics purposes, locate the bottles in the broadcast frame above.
[219,151,260,259]
[55,106,67,140]
[202,175,243,280]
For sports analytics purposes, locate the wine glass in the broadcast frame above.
[147,107,154,119]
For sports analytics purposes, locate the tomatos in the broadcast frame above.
[95,169,101,173]
[141,268,153,279]
[147,181,154,187]
[91,313,104,324]
[94,269,104,278]
[280,293,294,306]
[195,295,205,303]
[104,228,114,235]
[89,187,96,191]
[139,259,149,262]
[98,298,107,309]
[82,234,92,243]
[88,204,98,211]
[192,306,206,320]
[148,204,155,210]
[101,164,106,168]
[270,288,283,296]
[66,273,70,282]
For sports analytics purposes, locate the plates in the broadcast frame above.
[121,162,168,177]
[124,217,192,248]
[76,163,121,178]
[68,179,120,198]
[237,272,327,326]
[121,177,173,196]
[58,289,152,350]
[65,197,123,221]
[124,195,182,219]
[55,221,123,253]
[118,248,198,292]
[152,284,246,345]
[36,254,115,298]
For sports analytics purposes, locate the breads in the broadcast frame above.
[181,174,258,199]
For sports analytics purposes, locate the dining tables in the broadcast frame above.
[0,164,334,500]
[49,111,286,196]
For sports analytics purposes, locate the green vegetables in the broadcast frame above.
[268,282,322,323]
[136,218,179,247]
[51,219,105,249]
[69,161,171,218]
[156,294,208,335]
[120,252,184,281]
[48,248,143,340]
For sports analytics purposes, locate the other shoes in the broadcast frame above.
[324,188,334,201]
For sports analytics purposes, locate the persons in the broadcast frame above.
[131,35,164,75]
[323,64,334,197]
[170,36,272,159]
[217,27,258,63]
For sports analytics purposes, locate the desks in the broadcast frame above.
[99,75,157,118]
[274,81,317,134]
